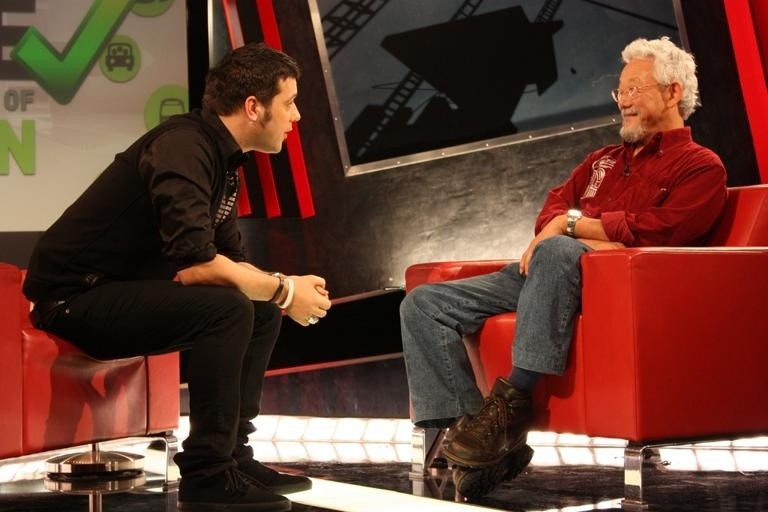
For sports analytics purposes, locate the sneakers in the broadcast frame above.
[176,467,292,512]
[450,415,535,499]
[441,374,534,470]
[236,457,313,495]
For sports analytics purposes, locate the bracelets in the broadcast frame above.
[268,271,295,310]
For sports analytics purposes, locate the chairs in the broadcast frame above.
[403,183,768,511]
[0,261,182,492]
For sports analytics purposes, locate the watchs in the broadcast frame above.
[565,208,582,237]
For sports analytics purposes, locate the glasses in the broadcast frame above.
[611,82,660,103]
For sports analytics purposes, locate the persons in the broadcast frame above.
[21,41,336,511]
[394,34,729,500]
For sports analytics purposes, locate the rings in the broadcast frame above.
[308,316,319,326]
